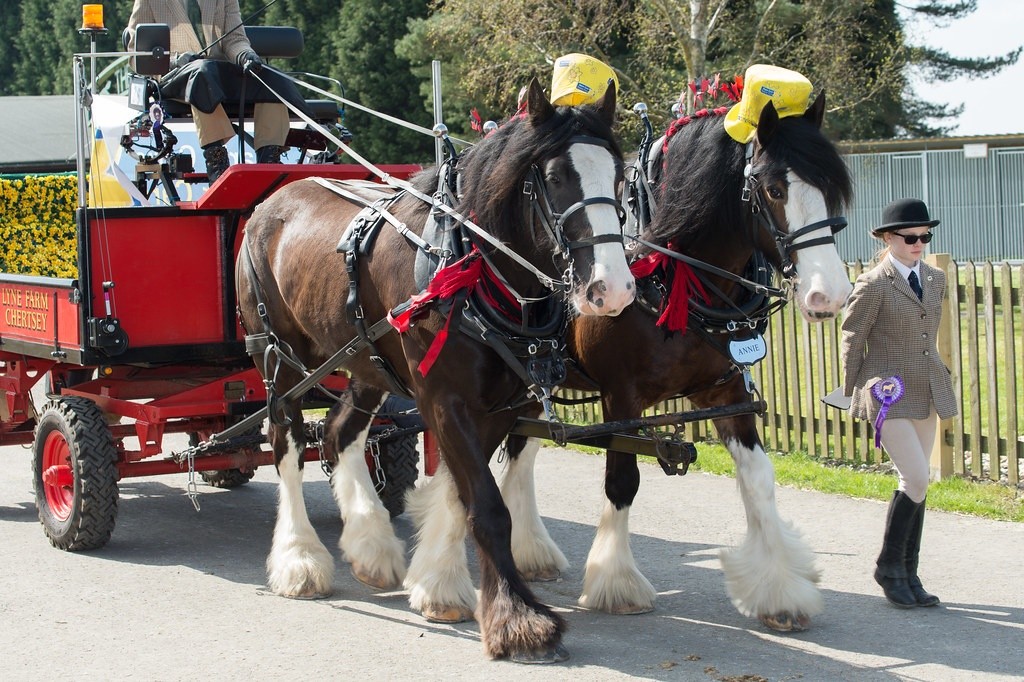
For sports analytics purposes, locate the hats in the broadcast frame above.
[724,64,813,144]
[550,53,619,107]
[872,198,941,238]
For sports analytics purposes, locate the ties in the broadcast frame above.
[907,270,922,302]
[187,0,207,55]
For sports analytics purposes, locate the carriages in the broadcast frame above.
[0,2,858,669]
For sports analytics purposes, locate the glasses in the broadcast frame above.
[893,227,934,245]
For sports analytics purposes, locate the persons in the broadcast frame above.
[840,198,959,608]
[127,0,290,188]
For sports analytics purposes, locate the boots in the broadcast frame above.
[256,145,283,164]
[203,146,230,188]
[874,490,940,609]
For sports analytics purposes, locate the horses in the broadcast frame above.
[231,75,640,664]
[498,88,858,635]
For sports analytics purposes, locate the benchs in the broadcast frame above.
[121,24,346,125]
